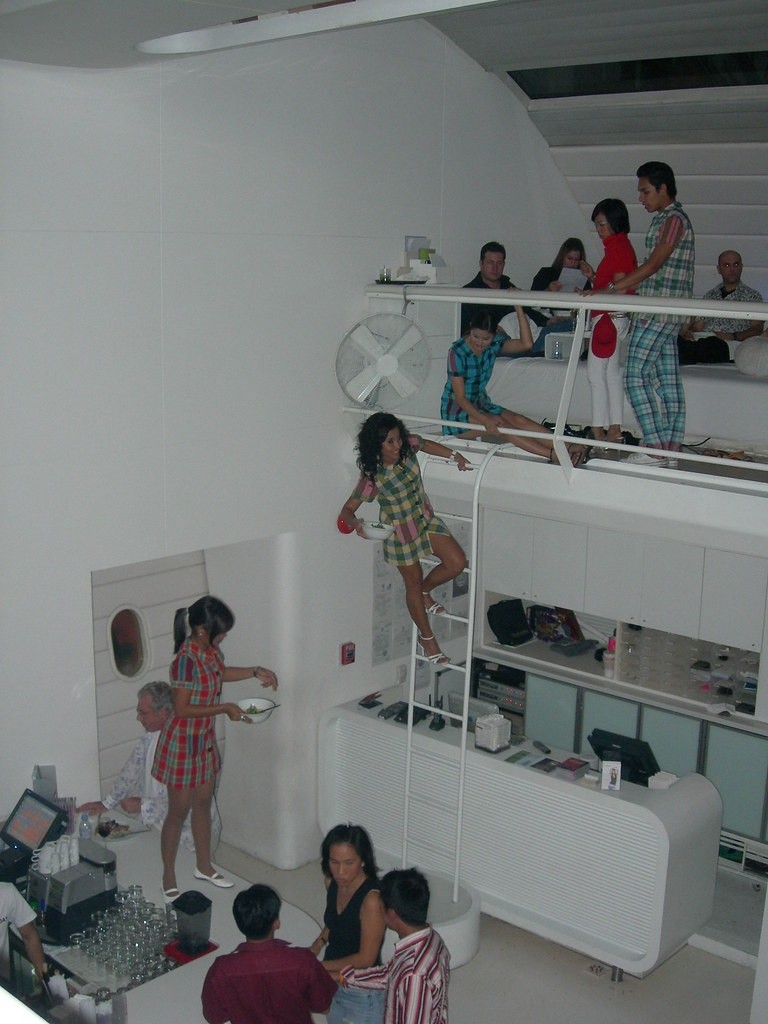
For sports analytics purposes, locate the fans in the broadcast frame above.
[335,313,433,403]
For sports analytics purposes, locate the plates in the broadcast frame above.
[95,821,138,841]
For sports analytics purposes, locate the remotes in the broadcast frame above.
[533,740,551,754]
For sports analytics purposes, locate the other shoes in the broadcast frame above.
[586,432,629,460]
[193,867,235,888]
[161,880,180,904]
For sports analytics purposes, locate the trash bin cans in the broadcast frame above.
[172,890,212,955]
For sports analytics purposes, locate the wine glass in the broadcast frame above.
[98,817,113,850]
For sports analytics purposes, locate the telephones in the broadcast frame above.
[430,696,445,731]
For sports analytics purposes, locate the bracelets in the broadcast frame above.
[253,666,260,676]
[449,450,457,460]
[732,333,737,340]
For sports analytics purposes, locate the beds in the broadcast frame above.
[470,313,768,454]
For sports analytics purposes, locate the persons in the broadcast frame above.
[341,412,473,666]
[201,883,338,1024]
[74,681,223,862]
[574,198,639,451]
[440,287,595,466]
[308,823,389,1024]
[0,881,47,986]
[460,241,573,357]
[530,237,592,317]
[677,249,765,366]
[575,161,694,468]
[609,768,618,790]
[150,596,279,904]
[328,867,451,1024]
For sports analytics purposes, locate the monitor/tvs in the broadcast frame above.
[0,789,70,858]
[587,729,661,788]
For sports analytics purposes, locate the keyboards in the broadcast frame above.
[648,770,679,786]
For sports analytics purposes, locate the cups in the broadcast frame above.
[551,341,563,360]
[603,650,616,679]
[31,834,80,874]
[70,884,178,1003]
[378,265,392,283]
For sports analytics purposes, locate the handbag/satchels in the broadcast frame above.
[487,598,533,646]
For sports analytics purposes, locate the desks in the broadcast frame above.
[544,331,591,361]
[320,700,724,983]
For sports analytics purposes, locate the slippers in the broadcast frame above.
[668,458,678,468]
[620,451,669,468]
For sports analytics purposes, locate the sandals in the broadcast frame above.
[417,633,450,666]
[567,442,591,455]
[550,449,584,468]
[421,592,447,615]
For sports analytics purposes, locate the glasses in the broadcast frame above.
[720,263,742,269]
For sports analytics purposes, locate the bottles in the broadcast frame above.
[607,628,616,651]
[79,815,92,840]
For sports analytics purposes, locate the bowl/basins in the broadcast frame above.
[238,697,275,724]
[692,332,716,341]
[361,521,396,543]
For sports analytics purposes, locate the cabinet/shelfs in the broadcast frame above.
[698,552,768,652]
[638,705,768,840]
[478,510,589,611]
[582,526,704,640]
[521,672,640,757]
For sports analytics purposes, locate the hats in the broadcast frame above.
[591,312,617,358]
